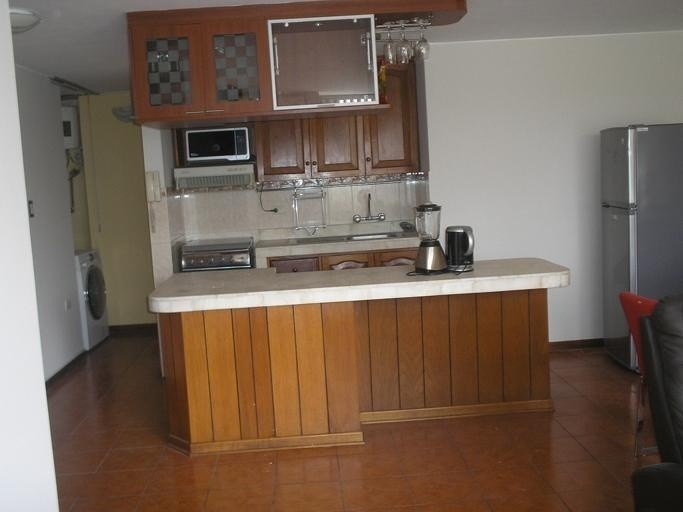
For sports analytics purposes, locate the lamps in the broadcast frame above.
[10,4,40,33]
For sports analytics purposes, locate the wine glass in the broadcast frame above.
[383,19,432,66]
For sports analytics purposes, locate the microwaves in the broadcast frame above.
[183,127,250,164]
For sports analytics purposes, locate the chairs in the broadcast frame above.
[615,287,683,512]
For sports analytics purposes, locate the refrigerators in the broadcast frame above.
[600,121,683,376]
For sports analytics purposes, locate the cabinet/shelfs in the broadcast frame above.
[365,55,419,178]
[126,4,272,126]
[253,110,365,181]
[318,247,420,271]
[268,256,320,273]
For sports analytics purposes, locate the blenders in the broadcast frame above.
[414,201,448,275]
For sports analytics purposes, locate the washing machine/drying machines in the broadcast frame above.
[75,250,110,351]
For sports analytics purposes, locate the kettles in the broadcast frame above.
[444,226,475,273]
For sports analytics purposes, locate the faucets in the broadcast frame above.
[367,192,372,218]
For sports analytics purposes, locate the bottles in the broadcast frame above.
[400,172,429,232]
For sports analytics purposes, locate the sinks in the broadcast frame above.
[346,235,394,240]
